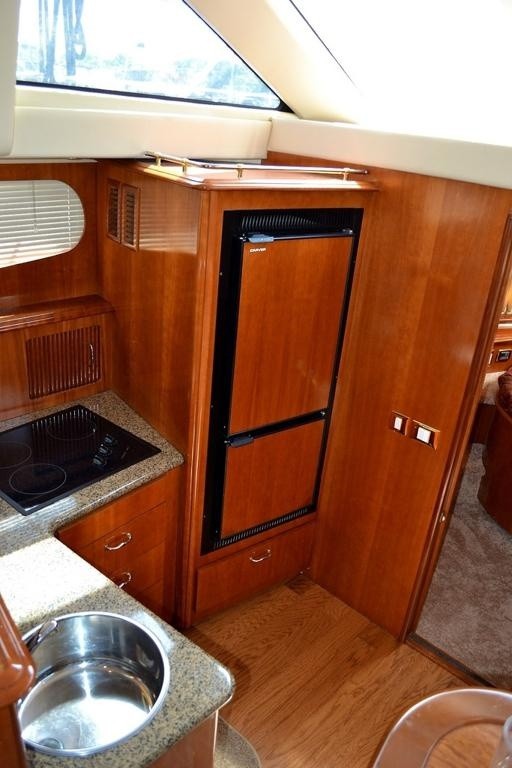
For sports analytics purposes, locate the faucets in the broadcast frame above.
[26,620,59,652]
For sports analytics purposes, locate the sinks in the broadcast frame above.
[16,610,170,757]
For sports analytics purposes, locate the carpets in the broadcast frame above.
[416,441,509,694]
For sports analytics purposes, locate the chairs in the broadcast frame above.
[477,397,511,532]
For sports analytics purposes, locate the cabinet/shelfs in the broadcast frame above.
[177,524,315,628]
[56,467,182,624]
[26,324,101,401]
[102,166,381,558]
